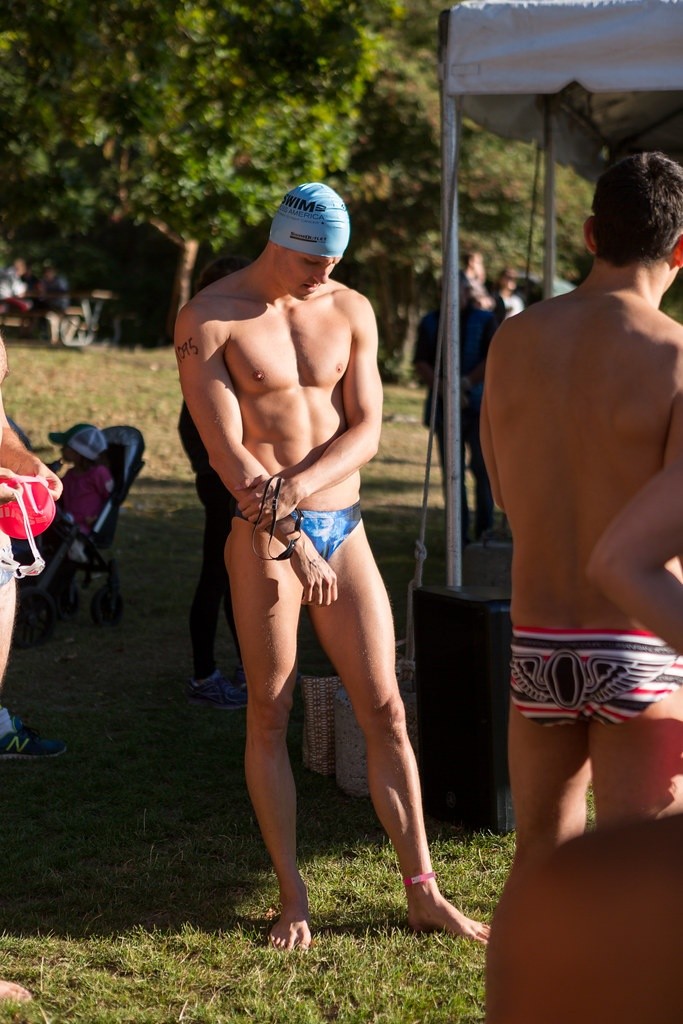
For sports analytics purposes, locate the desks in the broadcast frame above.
[26,289,122,346]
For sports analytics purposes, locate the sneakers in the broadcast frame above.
[187,674,248,709]
[0,716,67,758]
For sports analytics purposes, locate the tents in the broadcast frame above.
[438,1,683,591]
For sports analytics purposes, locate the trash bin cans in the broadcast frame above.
[296,635,348,775]
[411,583,518,834]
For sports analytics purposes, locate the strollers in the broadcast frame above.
[12,425,147,649]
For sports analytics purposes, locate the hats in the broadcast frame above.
[270,182,349,257]
[48,422,109,460]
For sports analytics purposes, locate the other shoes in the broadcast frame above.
[463,534,491,548]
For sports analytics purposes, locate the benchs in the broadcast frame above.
[0,304,86,346]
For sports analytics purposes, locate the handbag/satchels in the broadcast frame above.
[301,673,345,775]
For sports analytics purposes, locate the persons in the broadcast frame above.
[0,333,64,1008]
[586,456,683,656]
[480,152,683,870]
[0,259,70,339]
[47,423,115,537]
[174,180,492,956]
[413,251,527,554]
[486,807,683,1024]
[179,216,274,713]
